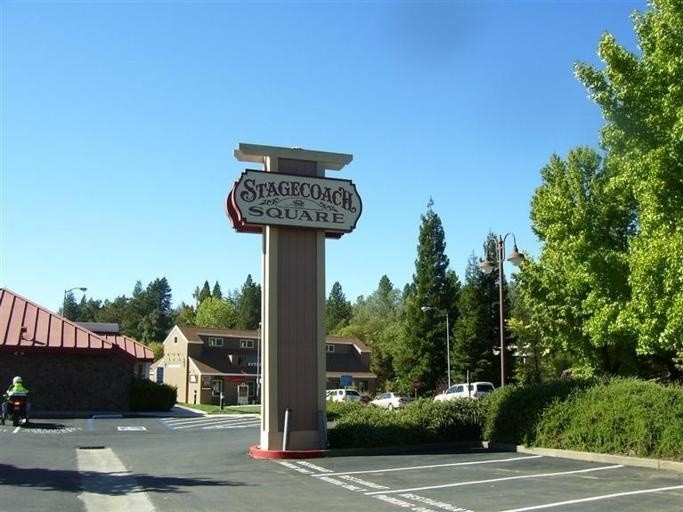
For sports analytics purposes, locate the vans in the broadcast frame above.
[433,381,497,403]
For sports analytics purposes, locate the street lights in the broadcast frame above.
[478,233,524,388]
[61,287,87,316]
[421,306,451,389]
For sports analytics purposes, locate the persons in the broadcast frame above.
[3,375,31,427]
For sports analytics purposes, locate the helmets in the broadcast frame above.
[13,376,22,386]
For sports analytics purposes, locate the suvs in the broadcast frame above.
[325,389,362,404]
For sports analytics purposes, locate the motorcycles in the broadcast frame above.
[3,393,28,426]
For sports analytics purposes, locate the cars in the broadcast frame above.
[368,392,414,411]
